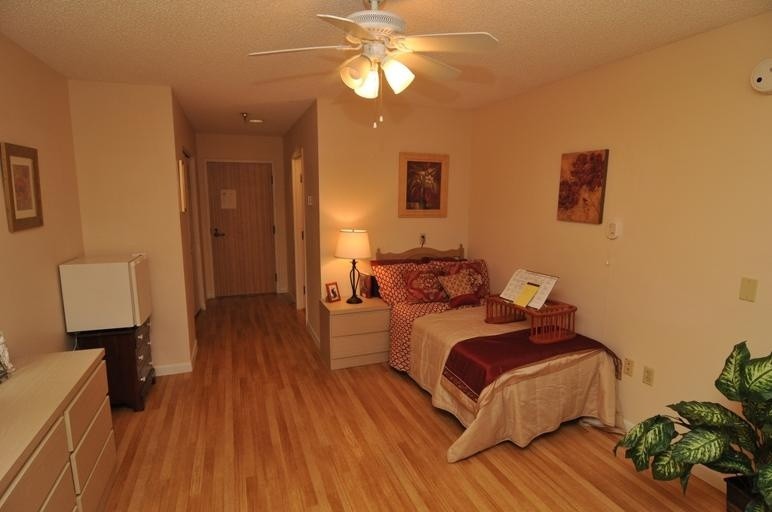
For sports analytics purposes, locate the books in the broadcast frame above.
[512,281,541,308]
[499,267,558,311]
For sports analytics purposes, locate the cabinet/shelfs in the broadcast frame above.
[1,347,119,512]
[65,317,158,413]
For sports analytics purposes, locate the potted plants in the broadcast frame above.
[612,335,771,511]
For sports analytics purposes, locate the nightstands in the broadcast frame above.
[317,294,391,373]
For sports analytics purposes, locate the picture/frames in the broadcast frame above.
[399,151,450,219]
[325,280,341,303]
[1,142,45,234]
[179,159,189,214]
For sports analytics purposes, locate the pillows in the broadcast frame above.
[367,254,492,311]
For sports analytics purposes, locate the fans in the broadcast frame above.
[239,0,502,86]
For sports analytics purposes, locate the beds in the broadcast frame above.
[374,240,624,463]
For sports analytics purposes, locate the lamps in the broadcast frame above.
[332,40,415,132]
[332,226,373,305]
